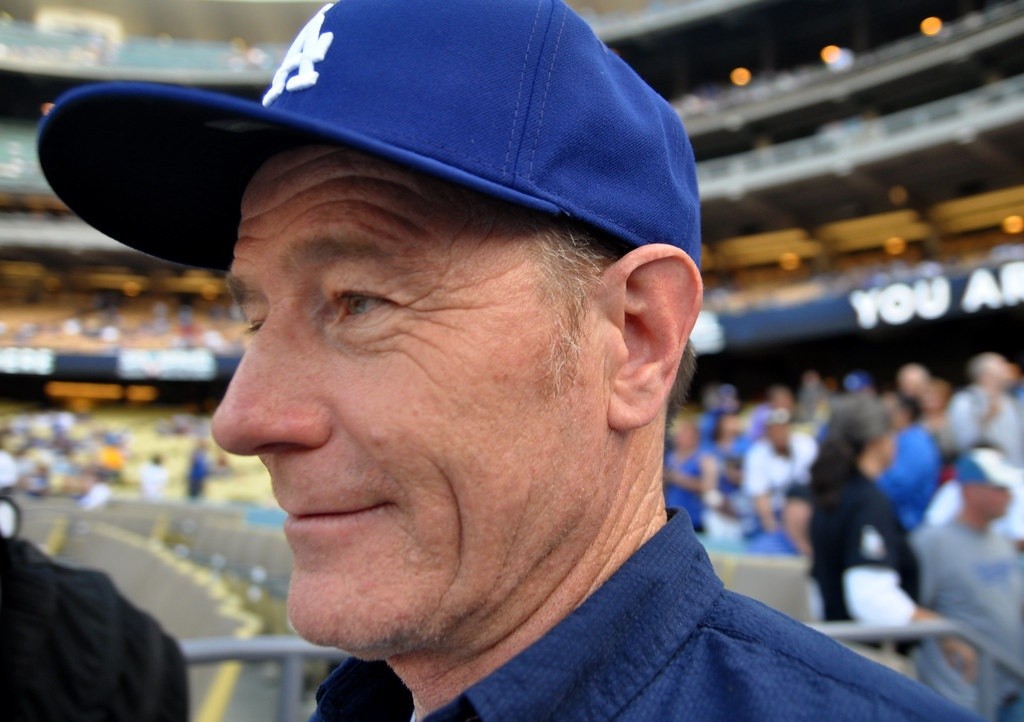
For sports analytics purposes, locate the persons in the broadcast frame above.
[1,1,1023,722]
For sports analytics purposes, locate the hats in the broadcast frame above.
[955,447,1021,486]
[36,0,702,273]
